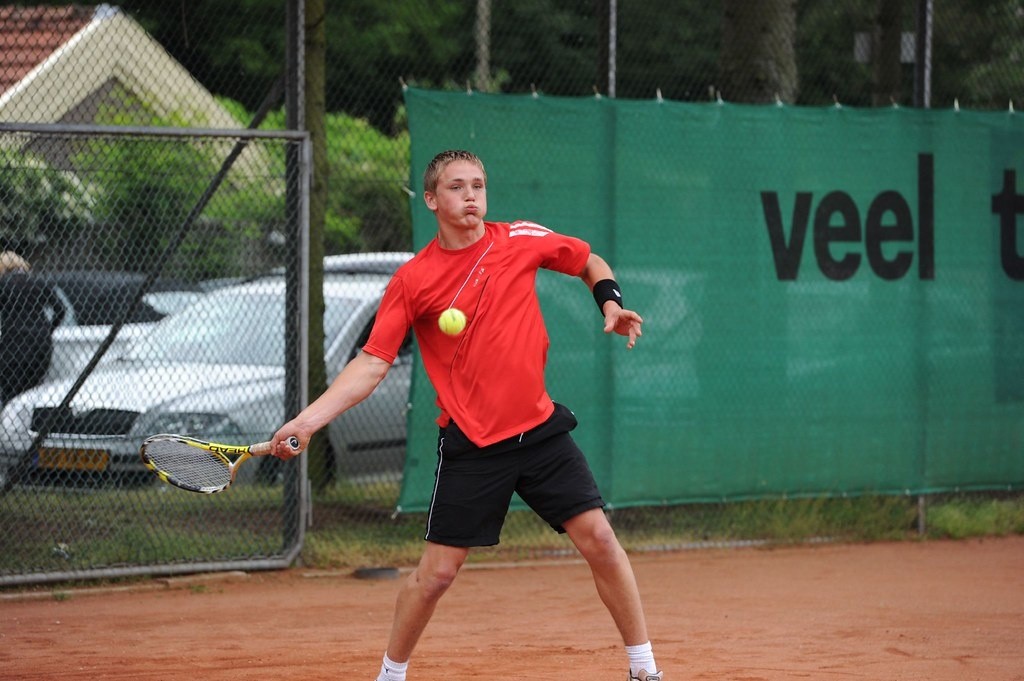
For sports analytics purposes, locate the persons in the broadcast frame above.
[266,148,661,680]
[0,250,67,411]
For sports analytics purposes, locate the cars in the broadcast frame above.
[2,238,420,506]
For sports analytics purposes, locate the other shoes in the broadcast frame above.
[626,670,664,680]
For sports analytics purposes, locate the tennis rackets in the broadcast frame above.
[138,433,299,495]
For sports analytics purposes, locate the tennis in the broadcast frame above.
[436,305,469,336]
